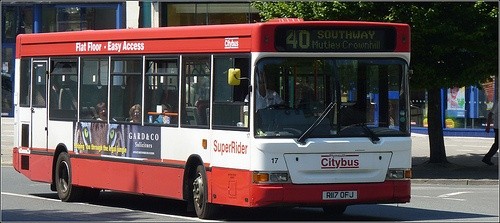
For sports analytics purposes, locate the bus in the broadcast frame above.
[13,21,412,220]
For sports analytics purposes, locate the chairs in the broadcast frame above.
[30,70,181,119]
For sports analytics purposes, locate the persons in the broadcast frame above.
[243,74,287,113]
[449,87,459,111]
[95,103,118,122]
[129,104,142,123]
[153,103,172,124]
[481,102,499,165]
[297,86,322,118]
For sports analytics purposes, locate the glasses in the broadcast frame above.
[259,79,266,84]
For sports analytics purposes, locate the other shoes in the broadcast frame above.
[481,157,495,166]
